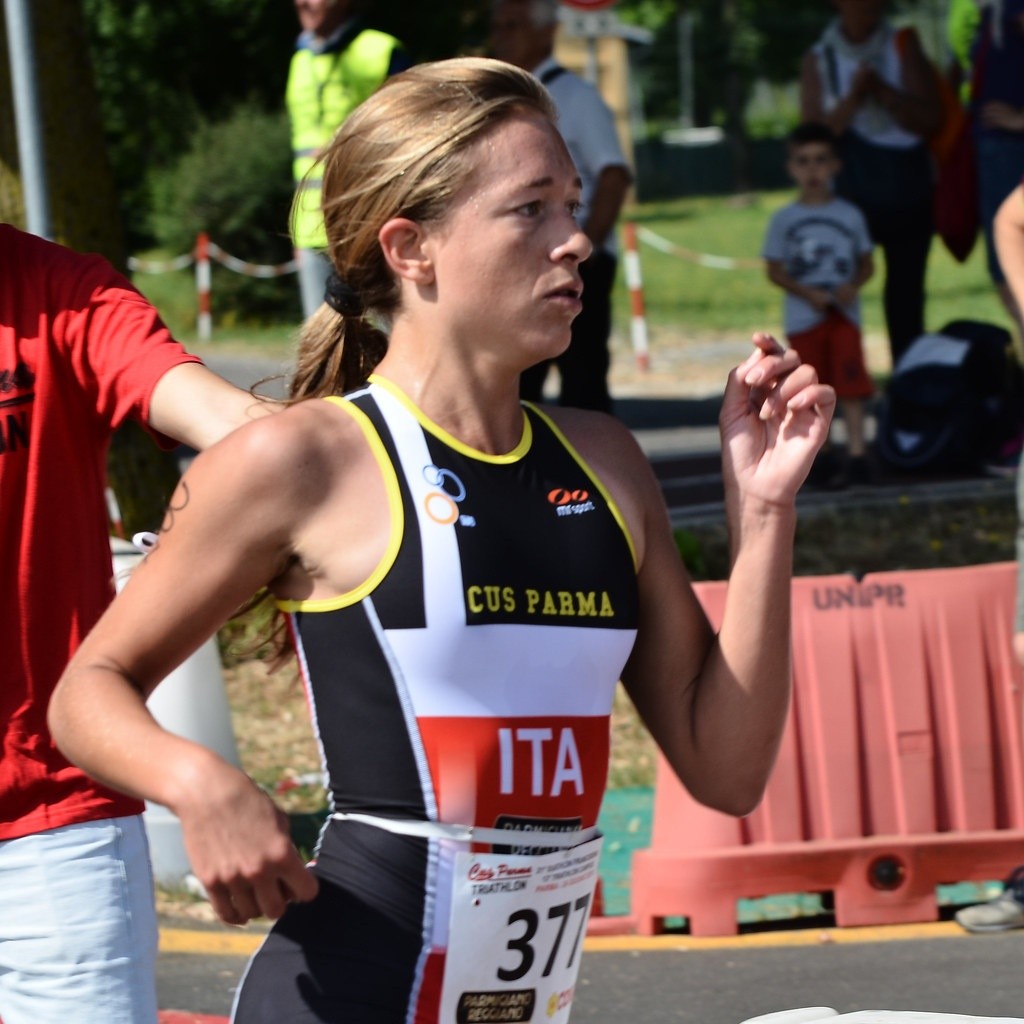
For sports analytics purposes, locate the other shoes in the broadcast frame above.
[836,447,867,478]
[957,889,1024,934]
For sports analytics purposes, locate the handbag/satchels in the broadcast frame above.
[893,26,977,264]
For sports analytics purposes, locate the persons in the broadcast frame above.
[47,57,837,1024]
[284,0,1024,940]
[0,224,287,1024]
